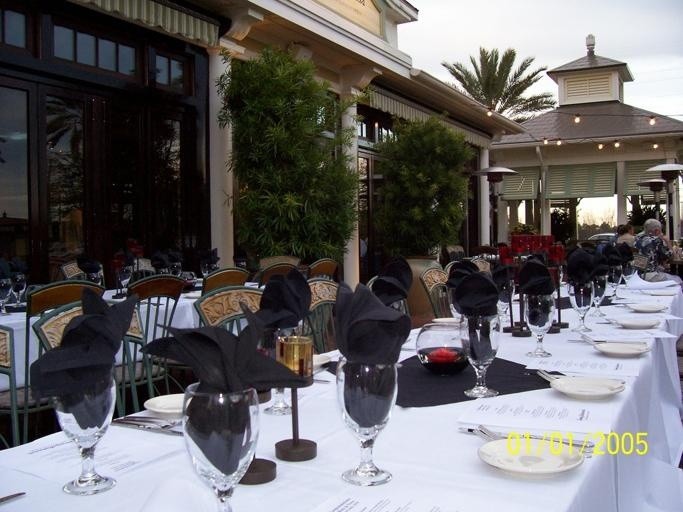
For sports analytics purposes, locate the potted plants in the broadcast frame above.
[368,110,475,322]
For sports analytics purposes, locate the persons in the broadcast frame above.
[609,225,624,247]
[617,224,636,249]
[633,219,683,291]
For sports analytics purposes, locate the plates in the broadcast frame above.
[617,317,660,329]
[311,353,332,371]
[478,438,586,477]
[594,342,651,358]
[627,303,668,312]
[550,378,624,399]
[431,316,462,324]
[143,393,196,414]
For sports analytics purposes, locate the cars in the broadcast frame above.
[586,232,616,242]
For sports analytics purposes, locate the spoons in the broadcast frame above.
[124,417,183,430]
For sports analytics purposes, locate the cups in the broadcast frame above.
[274,335,312,380]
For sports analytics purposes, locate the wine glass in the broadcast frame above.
[460,315,503,398]
[497,283,516,322]
[567,283,593,333]
[593,276,606,317]
[523,294,556,358]
[333,353,400,487]
[0,259,248,318]
[180,379,261,512]
[605,266,624,314]
[458,259,632,399]
[51,365,117,498]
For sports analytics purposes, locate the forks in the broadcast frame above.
[470,425,594,457]
[537,369,626,385]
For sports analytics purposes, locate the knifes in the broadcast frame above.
[311,377,331,384]
[0,491,27,505]
[112,420,185,439]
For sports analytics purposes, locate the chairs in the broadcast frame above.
[0,237,682,511]
